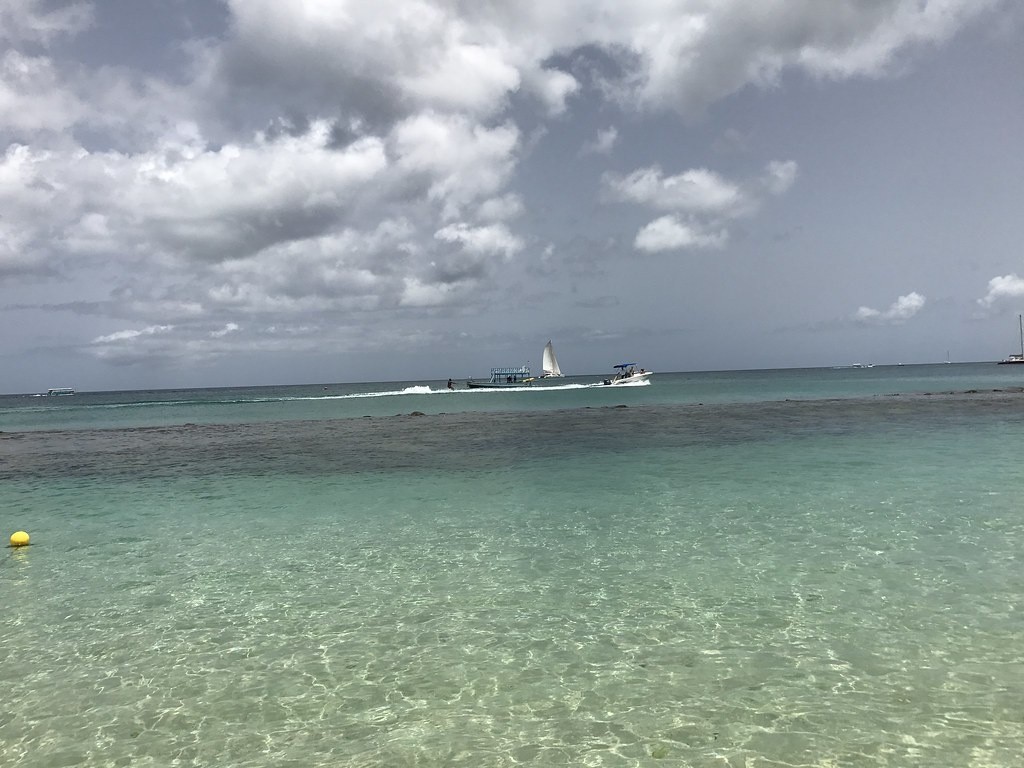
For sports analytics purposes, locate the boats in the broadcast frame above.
[466,367,553,389]
[997,312,1024,364]
[833,363,876,368]
[48,388,75,396]
[589,363,652,386]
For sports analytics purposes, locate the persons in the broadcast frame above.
[617,365,645,380]
[506,374,517,383]
[447,379,454,391]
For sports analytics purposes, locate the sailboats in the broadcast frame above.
[539,340,566,379]
